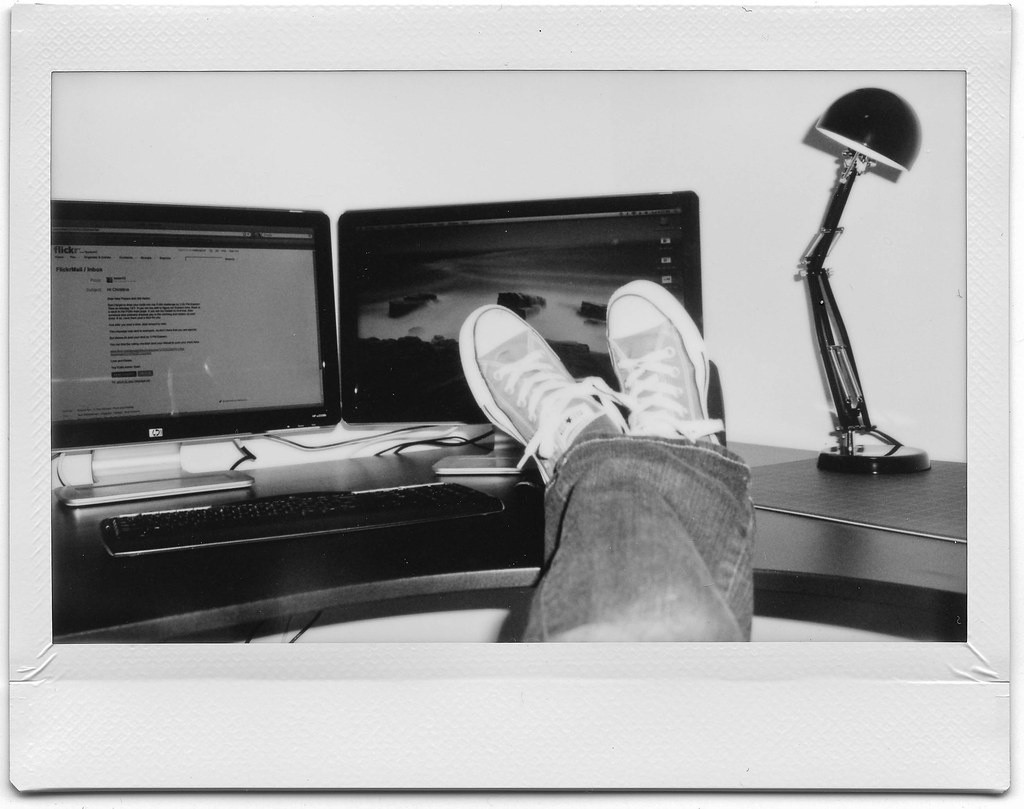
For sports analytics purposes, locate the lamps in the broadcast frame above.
[793,88,931,478]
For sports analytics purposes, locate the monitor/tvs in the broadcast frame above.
[49,198,341,507]
[337,191,705,475]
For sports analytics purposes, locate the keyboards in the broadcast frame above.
[99,482,506,556]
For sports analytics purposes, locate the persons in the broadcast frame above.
[458,278,757,642]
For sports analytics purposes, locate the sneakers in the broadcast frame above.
[605,280,724,446]
[459,304,622,486]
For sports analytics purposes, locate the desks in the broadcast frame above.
[51,445,966,643]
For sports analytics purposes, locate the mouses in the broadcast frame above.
[512,480,546,508]
